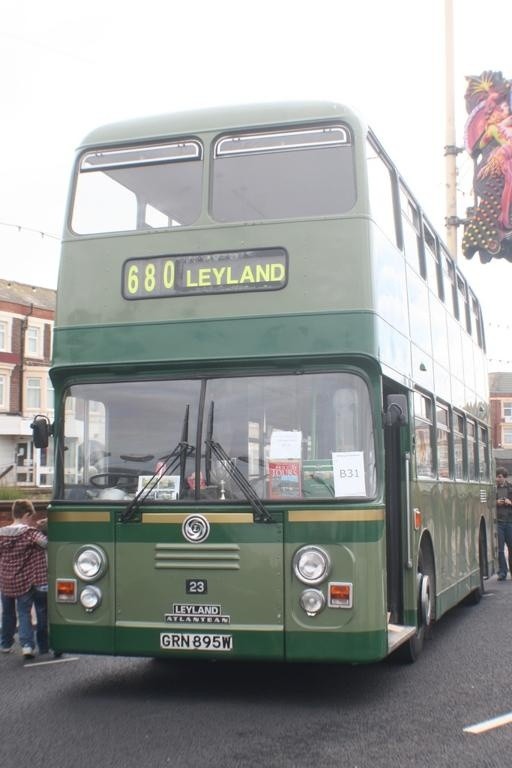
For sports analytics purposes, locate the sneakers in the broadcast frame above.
[38,645,62,657]
[1,647,14,654]
[20,643,36,657]
[497,572,506,581]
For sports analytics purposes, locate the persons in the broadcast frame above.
[495,466,511,582]
[31,518,64,659]
[0,500,48,659]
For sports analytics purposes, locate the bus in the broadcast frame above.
[31,99,494,662]
